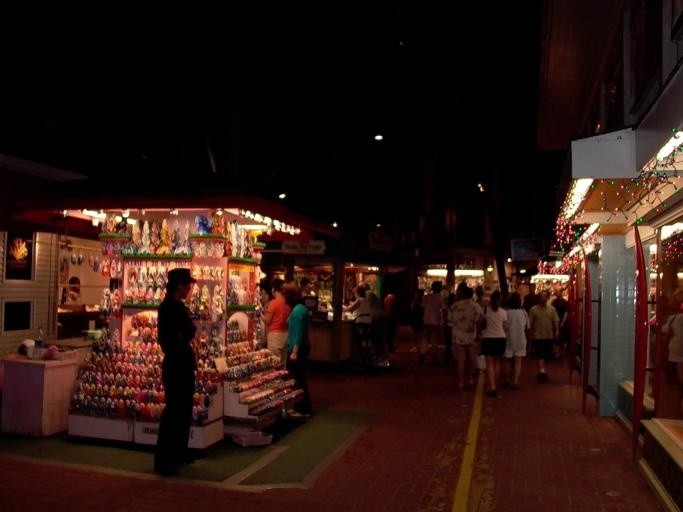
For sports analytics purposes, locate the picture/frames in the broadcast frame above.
[3,231,36,283]
[1,298,34,334]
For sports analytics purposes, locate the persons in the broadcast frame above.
[257,277,290,367]
[342,278,568,400]
[100,279,118,309]
[58,275,80,305]
[299,277,317,297]
[282,283,312,419]
[151,268,201,480]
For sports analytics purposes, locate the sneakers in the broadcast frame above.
[287,408,296,414]
[290,411,311,418]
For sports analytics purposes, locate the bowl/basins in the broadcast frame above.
[87,331,100,338]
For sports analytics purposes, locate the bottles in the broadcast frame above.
[32,326,44,359]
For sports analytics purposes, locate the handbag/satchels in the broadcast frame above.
[477,305,488,330]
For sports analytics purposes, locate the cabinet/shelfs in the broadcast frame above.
[0,354,77,437]
[59,207,311,449]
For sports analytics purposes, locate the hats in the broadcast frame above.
[164,268,197,286]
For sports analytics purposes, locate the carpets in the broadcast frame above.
[5,409,380,493]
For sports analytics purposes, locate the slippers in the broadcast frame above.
[485,390,496,397]
